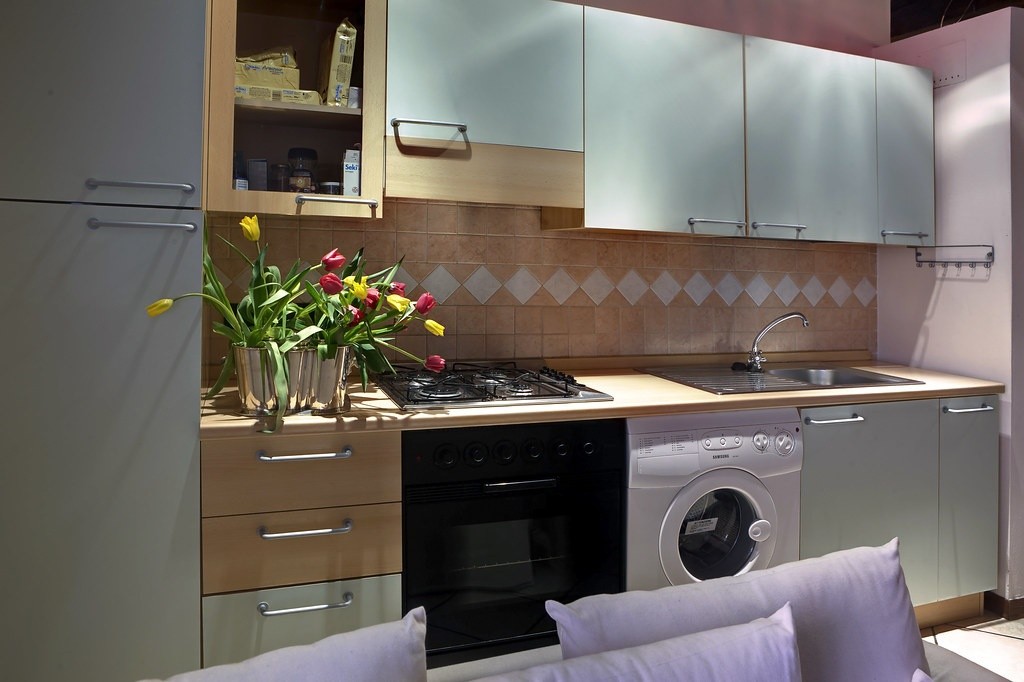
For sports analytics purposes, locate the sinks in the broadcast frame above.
[767,365,925,388]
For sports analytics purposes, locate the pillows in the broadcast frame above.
[545,537,931,682]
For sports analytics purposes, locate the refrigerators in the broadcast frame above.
[0,0,206,682]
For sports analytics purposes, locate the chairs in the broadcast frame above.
[142,605,428,681]
[473,602,804,680]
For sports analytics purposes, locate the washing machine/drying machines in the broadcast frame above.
[624,407,804,592]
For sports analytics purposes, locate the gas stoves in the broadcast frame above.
[362,357,614,411]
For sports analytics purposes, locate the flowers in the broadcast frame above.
[146,214,447,398]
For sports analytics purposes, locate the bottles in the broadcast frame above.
[275,164,292,191]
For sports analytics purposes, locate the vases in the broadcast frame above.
[231,344,354,418]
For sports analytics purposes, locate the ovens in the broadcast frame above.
[401,418,628,658]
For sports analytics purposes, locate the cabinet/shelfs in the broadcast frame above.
[202,1,935,251]
[199,426,403,671]
[800,394,1002,628]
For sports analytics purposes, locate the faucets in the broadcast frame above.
[747,311,810,371]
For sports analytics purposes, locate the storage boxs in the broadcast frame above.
[331,149,360,196]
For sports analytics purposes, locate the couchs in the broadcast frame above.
[425,644,1010,681]
